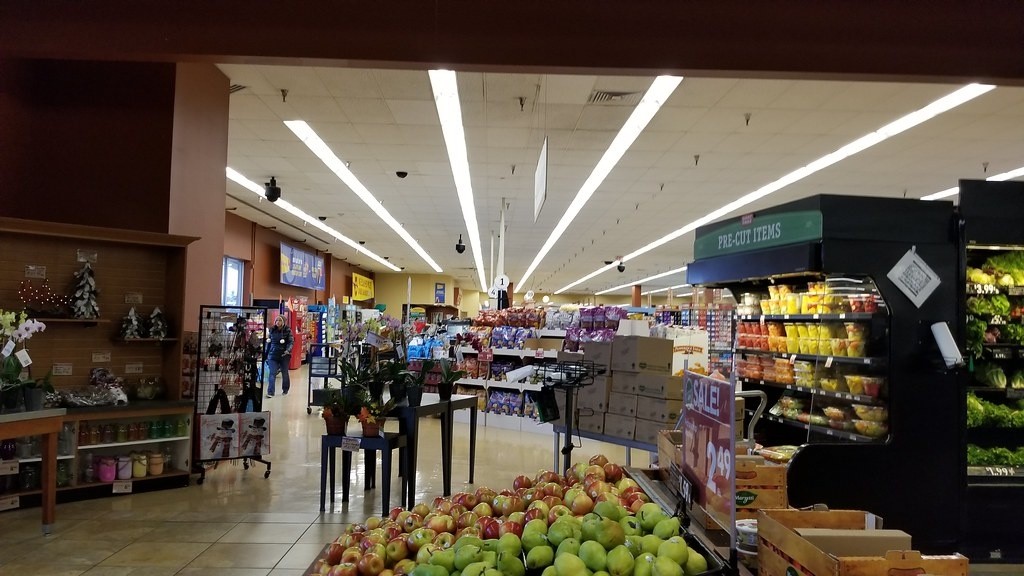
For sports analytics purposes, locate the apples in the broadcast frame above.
[449,453,650,539]
[307,496,457,576]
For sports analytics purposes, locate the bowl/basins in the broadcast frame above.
[851,419,888,436]
[763,446,799,464]
[778,399,829,426]
[823,407,851,420]
[852,403,887,420]
[829,421,855,430]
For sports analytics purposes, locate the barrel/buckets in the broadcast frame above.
[98,454,164,482]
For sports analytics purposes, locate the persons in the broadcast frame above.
[265,314,293,399]
[412,318,425,333]
[239,329,263,413]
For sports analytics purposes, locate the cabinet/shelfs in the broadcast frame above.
[718,287,889,437]
[29,317,179,342]
[951,247,1024,483]
[421,327,564,390]
[306,342,364,413]
[0,407,199,512]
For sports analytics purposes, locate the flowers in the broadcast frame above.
[0,307,46,356]
[323,395,346,421]
[355,394,394,423]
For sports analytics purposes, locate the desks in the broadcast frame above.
[333,399,449,503]
[371,394,484,483]
[320,432,410,517]
[0,406,67,538]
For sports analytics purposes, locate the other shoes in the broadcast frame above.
[266,393,275,397]
[282,392,288,396]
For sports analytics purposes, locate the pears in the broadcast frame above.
[409,500,709,576]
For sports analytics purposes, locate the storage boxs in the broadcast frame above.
[311,386,341,404]
[550,321,968,576]
[313,356,345,377]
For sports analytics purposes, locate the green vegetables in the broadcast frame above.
[962,253,1024,467]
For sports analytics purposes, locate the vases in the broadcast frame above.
[325,418,349,436]
[362,417,386,435]
[0,356,23,380]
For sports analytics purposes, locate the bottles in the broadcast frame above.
[18,465,40,494]
[79,418,185,447]
[428,332,457,359]
[407,335,431,362]
[58,424,75,454]
[162,447,172,471]
[83,453,96,483]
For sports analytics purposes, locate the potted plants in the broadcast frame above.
[342,356,470,410]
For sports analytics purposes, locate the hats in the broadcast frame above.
[266,315,287,344]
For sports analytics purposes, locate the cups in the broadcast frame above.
[736,322,787,353]
[433,351,445,359]
[768,282,845,314]
[735,519,762,569]
[23,383,45,411]
[759,298,771,315]
[783,322,866,357]
[844,375,879,396]
[432,347,443,356]
[736,293,768,316]
